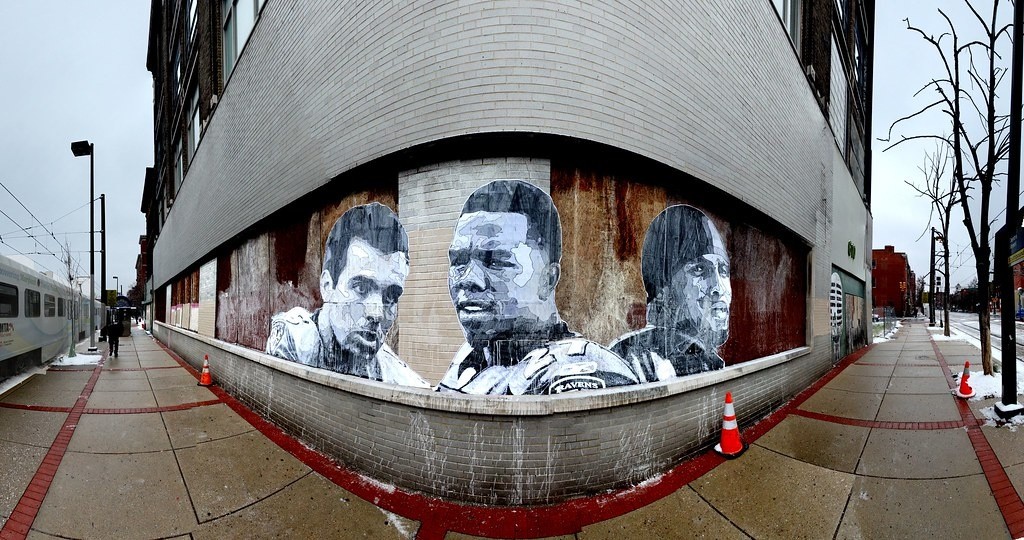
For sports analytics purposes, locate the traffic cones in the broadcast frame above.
[196,354,215,386]
[714,391,750,460]
[956,360,976,398]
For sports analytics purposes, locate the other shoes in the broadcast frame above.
[109,349,118,357]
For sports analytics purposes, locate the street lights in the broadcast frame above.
[70,139,98,352]
[114,276,119,303]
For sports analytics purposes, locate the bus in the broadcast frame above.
[0,254,112,381]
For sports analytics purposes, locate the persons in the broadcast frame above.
[108,318,120,357]
[135,315,138,323]
